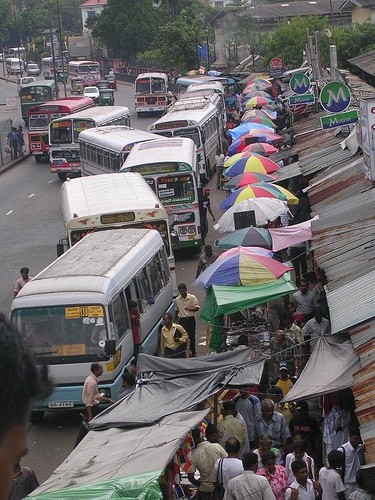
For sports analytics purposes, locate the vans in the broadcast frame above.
[27,64,41,75]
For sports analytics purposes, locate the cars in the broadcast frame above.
[84,86,99,103]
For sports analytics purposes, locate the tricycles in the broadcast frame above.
[97,80,109,92]
[71,77,84,94]
[105,74,116,90]
[55,70,67,82]
[98,91,115,106]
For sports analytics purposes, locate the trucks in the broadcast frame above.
[6,57,24,74]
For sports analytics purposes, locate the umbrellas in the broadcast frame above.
[243,115,277,128]
[243,76,273,94]
[227,122,276,140]
[223,152,263,167]
[216,247,274,261]
[212,197,289,234]
[243,96,273,108]
[222,156,280,177]
[214,227,272,250]
[222,172,277,189]
[241,108,277,121]
[245,91,271,99]
[241,142,278,155]
[228,129,282,155]
[192,253,295,292]
[218,182,299,208]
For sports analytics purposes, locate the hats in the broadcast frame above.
[204,188,210,193]
[278,364,287,371]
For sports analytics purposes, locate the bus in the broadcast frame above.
[50,106,133,178]
[68,60,99,87]
[183,81,225,93]
[19,80,61,127]
[29,95,95,164]
[9,230,175,424]
[168,94,225,104]
[79,125,163,175]
[56,171,178,295]
[119,137,204,253]
[148,106,224,182]
[177,75,234,84]
[137,73,166,118]
[42,56,66,80]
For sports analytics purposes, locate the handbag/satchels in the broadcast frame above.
[212,458,225,500]
[5,145,11,153]
[21,145,26,153]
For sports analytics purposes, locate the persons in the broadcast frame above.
[348,470,375,500]
[271,367,297,397]
[174,283,200,357]
[128,300,140,358]
[319,450,346,500]
[269,386,293,423]
[212,437,244,500]
[161,313,189,357]
[109,67,114,75]
[119,373,136,397]
[271,273,332,371]
[288,240,310,287]
[214,149,225,190]
[13,267,34,298]
[218,401,250,457]
[221,92,242,156]
[223,452,276,500]
[286,400,322,448]
[203,187,215,245]
[274,80,293,188]
[130,359,137,374]
[254,399,291,450]
[254,434,281,467]
[196,245,219,279]
[232,335,251,349]
[6,125,25,159]
[285,461,323,500]
[188,424,228,500]
[283,431,319,482]
[232,387,262,451]
[73,362,116,447]
[255,452,291,500]
[0,309,54,500]
[337,429,365,497]
